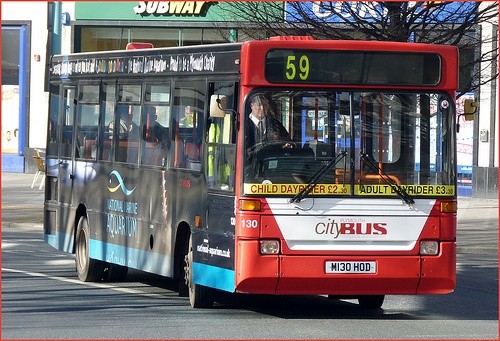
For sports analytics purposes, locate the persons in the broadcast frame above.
[246,91,295,157]
[109,104,164,140]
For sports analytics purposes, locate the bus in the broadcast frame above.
[42,34,481,312]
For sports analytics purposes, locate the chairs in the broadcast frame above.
[64,126,204,171]
